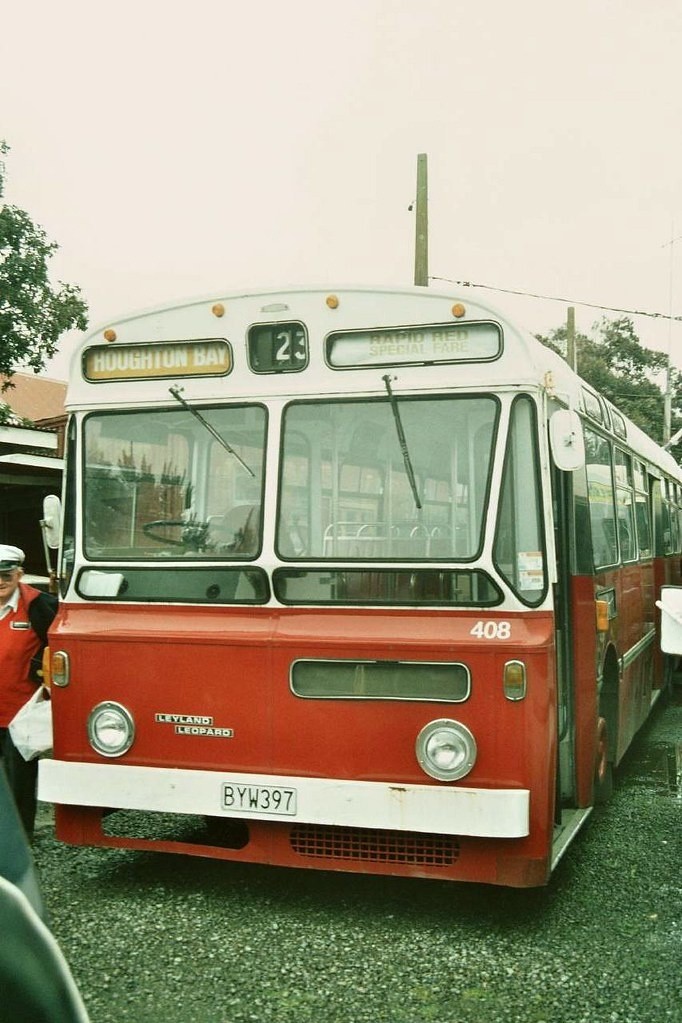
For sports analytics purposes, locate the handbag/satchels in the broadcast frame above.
[8,684,52,761]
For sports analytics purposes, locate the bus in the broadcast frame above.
[36,286,682,893]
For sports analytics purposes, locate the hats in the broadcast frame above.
[0,544,26,571]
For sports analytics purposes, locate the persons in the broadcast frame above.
[0,545,59,849]
